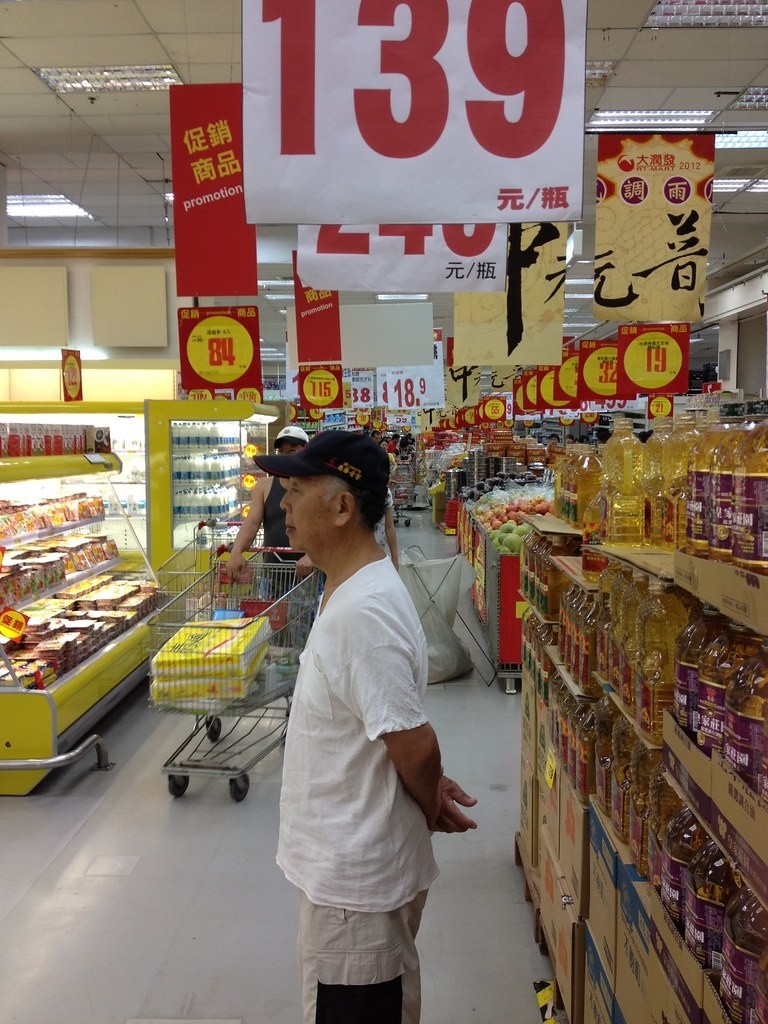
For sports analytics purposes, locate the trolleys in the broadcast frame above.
[144,544,324,804]
[388,472,416,527]
[156,518,263,646]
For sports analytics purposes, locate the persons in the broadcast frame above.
[255,430,477,1024]
[545,427,655,464]
[226,427,313,599]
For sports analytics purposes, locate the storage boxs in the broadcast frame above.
[434,427,565,464]
[518,553,768,1023]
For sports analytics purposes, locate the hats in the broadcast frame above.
[253,429,390,492]
[273,425,309,448]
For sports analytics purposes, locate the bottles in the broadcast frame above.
[523,399,768,1024]
[171,422,247,519]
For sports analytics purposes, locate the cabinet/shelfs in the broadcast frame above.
[269,398,467,537]
[460,504,523,677]
